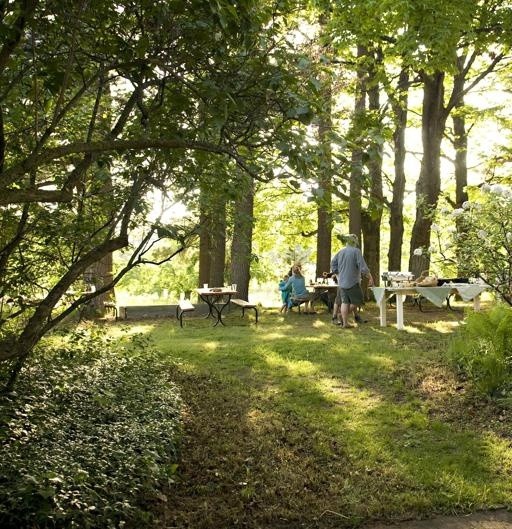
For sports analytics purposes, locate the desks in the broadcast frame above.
[368,285,503,330]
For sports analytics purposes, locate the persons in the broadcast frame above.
[278,234,374,328]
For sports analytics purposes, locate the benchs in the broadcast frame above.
[280,297,309,314]
[230,298,258,326]
[104,304,117,322]
[176,301,195,328]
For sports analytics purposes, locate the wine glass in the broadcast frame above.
[316,277,333,285]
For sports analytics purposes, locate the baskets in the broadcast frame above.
[415,270,438,286]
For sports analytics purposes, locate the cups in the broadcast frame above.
[227,286,231,291]
[91,286,95,292]
[204,283,208,290]
[468,276,484,285]
[309,279,312,284]
[232,284,238,292]
[180,293,185,301]
